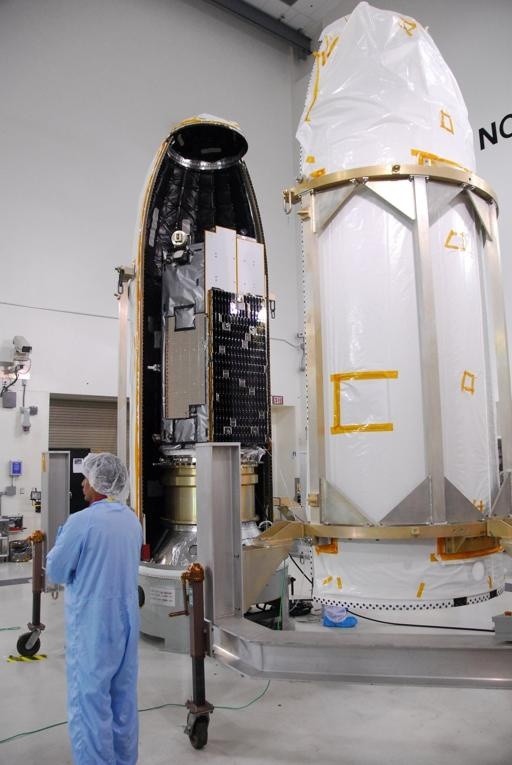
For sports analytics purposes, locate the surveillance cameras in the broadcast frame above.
[11,334,33,362]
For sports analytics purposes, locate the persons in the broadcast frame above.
[322,605,358,628]
[46,453,143,765]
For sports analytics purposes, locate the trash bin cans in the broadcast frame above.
[9,540,33,562]
